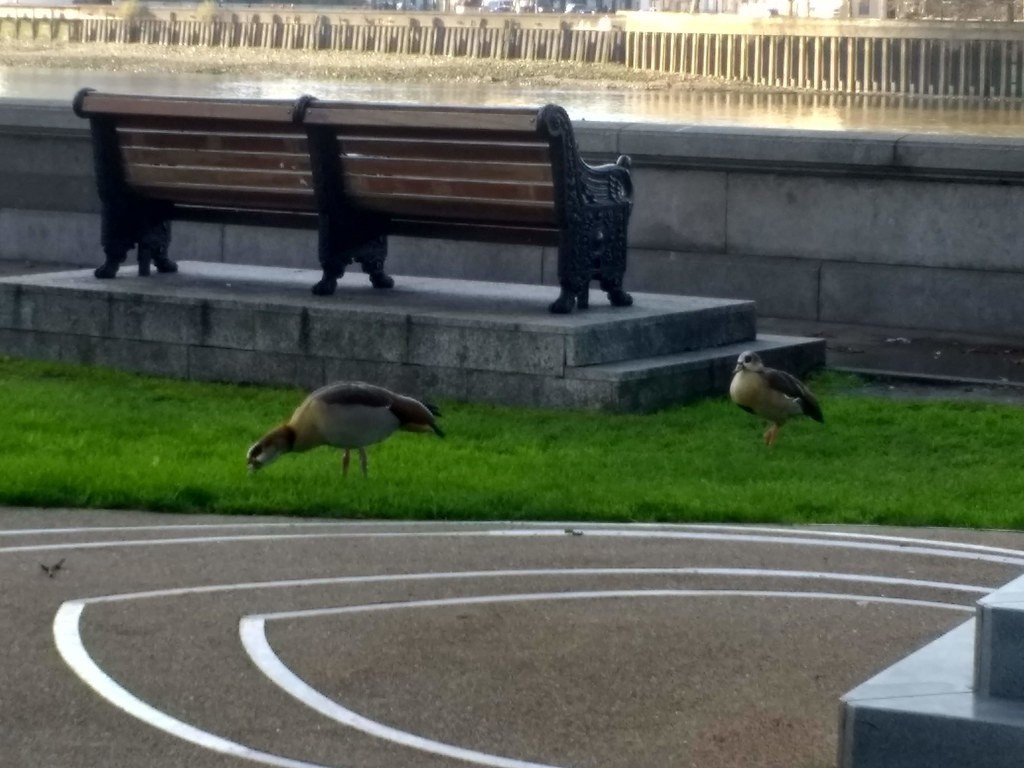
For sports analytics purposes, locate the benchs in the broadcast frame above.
[70,86,636,316]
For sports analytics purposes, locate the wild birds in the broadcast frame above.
[730,350,825,449]
[246,381,446,481]
